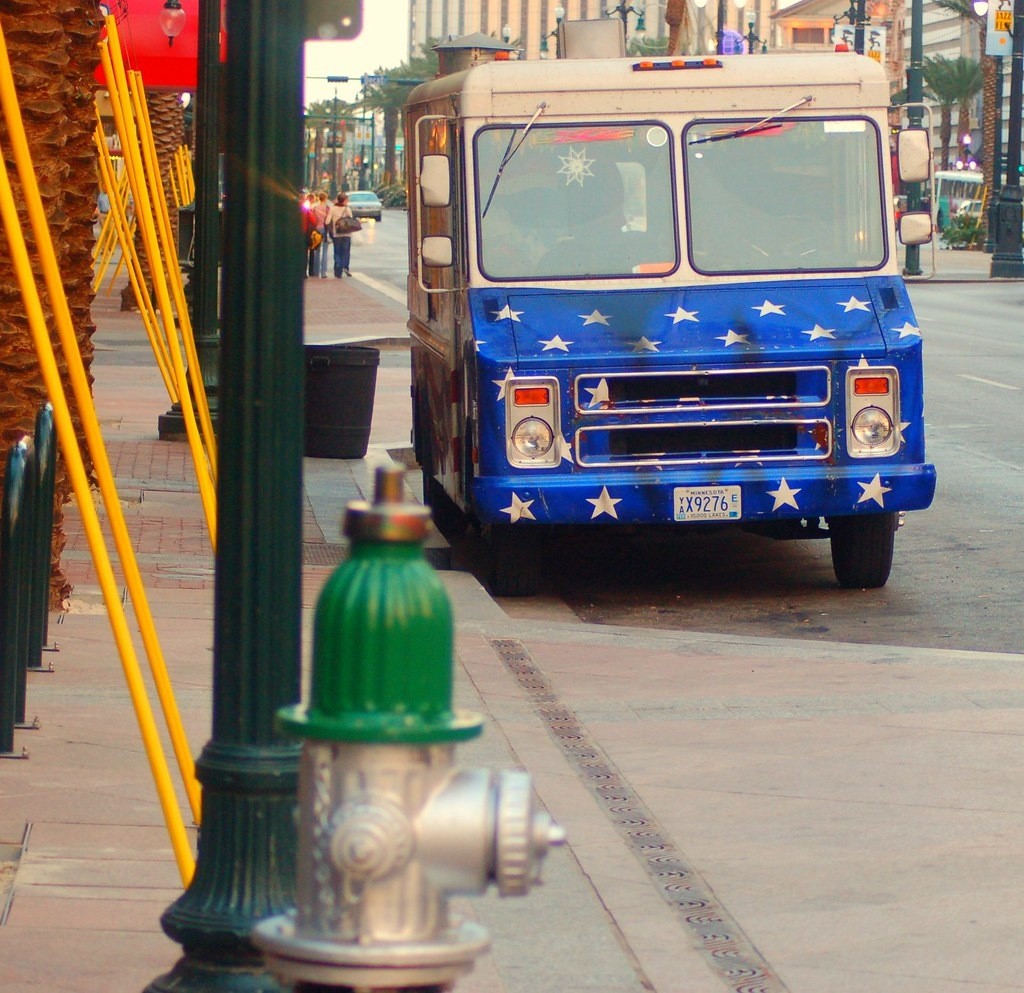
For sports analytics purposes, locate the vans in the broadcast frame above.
[403,54,936,597]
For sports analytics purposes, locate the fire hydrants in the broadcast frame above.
[247,459,571,993]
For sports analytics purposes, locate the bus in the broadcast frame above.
[932,170,1024,240]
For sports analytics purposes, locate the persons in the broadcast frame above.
[300,192,353,280]
[98,191,116,255]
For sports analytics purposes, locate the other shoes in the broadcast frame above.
[343,270,352,276]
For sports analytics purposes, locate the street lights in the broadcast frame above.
[538,3,565,60]
[605,0,646,57]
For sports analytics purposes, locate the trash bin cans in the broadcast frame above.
[304,344,380,460]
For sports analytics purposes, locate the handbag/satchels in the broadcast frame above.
[306,224,322,249]
[335,208,362,233]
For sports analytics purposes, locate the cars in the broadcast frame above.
[346,191,384,222]
